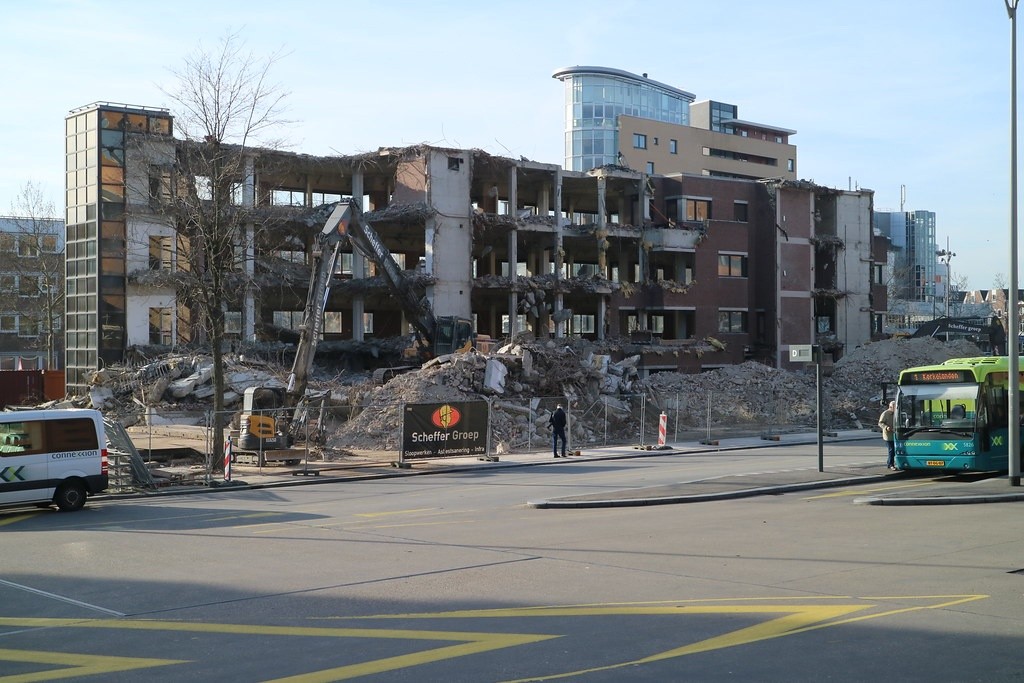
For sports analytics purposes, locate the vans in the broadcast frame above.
[0,407,109,514]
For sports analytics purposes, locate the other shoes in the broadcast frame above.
[561,455,567,457]
[554,455,560,458]
[890,466,897,470]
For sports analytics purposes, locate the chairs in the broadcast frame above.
[951,404,966,419]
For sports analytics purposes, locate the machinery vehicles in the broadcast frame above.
[224,192,498,465]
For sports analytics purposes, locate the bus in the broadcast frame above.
[893,353,1024,473]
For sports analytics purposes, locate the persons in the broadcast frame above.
[877,400,897,471]
[549,403,568,457]
